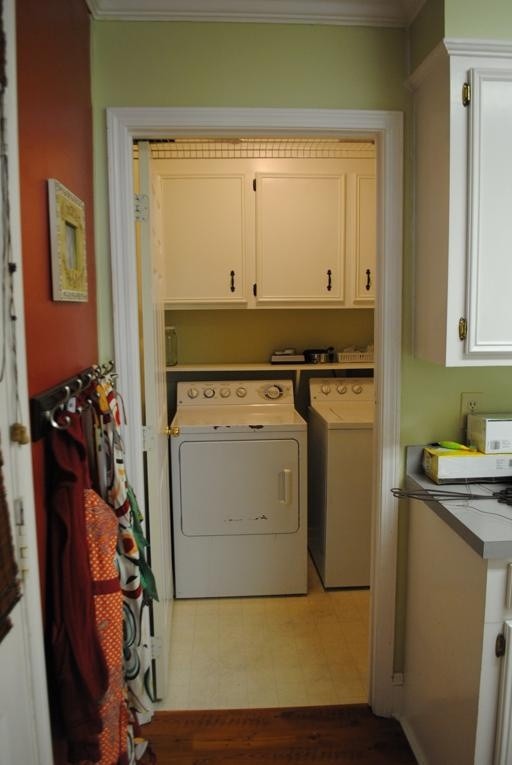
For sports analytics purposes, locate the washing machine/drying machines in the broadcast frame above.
[309,377,375,590]
[170,379,309,601]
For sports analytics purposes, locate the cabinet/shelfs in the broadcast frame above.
[152,157,377,309]
[407,39,512,366]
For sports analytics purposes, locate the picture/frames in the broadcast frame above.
[48,178,90,304]
